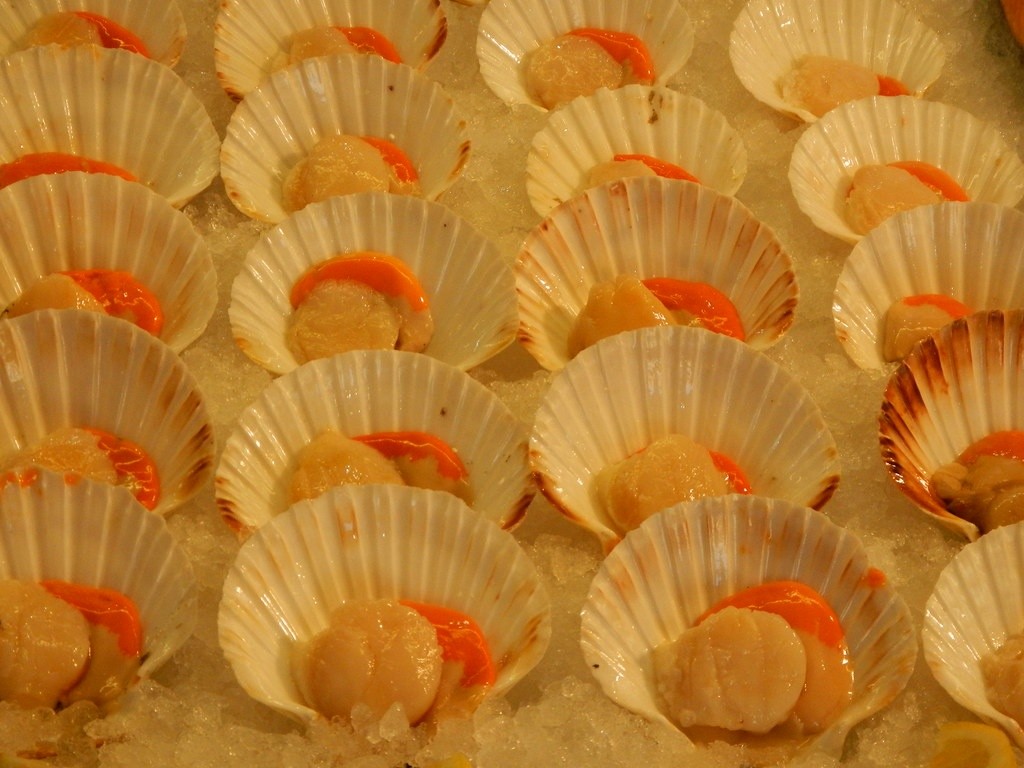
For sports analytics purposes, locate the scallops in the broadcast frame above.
[0,0,1024,756]
